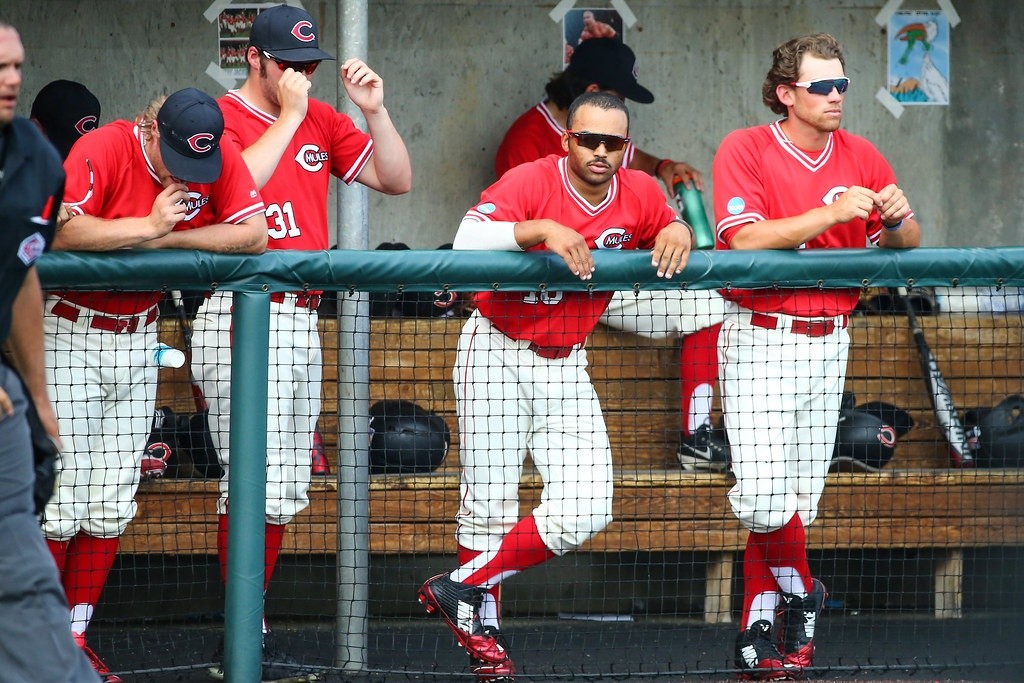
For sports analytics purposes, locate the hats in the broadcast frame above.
[570,37,654,105]
[250,3,337,62]
[31,80,101,155]
[157,88,224,183]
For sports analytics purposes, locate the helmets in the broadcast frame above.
[367,399,451,473]
[959,395,1024,468]
[827,395,915,473]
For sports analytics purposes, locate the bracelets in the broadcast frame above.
[883,210,914,231]
[654,159,673,177]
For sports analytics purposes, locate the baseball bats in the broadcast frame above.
[896,285,975,469]
[170,290,209,414]
[310,422,331,476]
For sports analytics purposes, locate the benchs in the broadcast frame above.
[109,318,1023,620]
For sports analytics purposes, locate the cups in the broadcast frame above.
[148,341,186,369]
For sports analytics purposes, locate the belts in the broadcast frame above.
[51,301,158,335]
[269,291,321,312]
[491,325,585,360]
[750,313,850,338]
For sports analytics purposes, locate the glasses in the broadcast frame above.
[787,77,851,96]
[252,44,320,75]
[565,129,632,152]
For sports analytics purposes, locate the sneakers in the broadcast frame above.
[675,426,734,474]
[417,572,509,664]
[732,619,794,681]
[464,625,517,682]
[778,578,829,676]
[77,642,122,683]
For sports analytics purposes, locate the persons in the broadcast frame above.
[190,4,413,683]
[34,87,270,683]
[579,10,616,40]
[27,78,101,164]
[493,38,733,472]
[710,33,921,683]
[417,90,694,683]
[0,20,106,682]
[218,10,255,37]
[220,43,248,66]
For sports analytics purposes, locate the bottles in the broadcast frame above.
[673,173,714,250]
